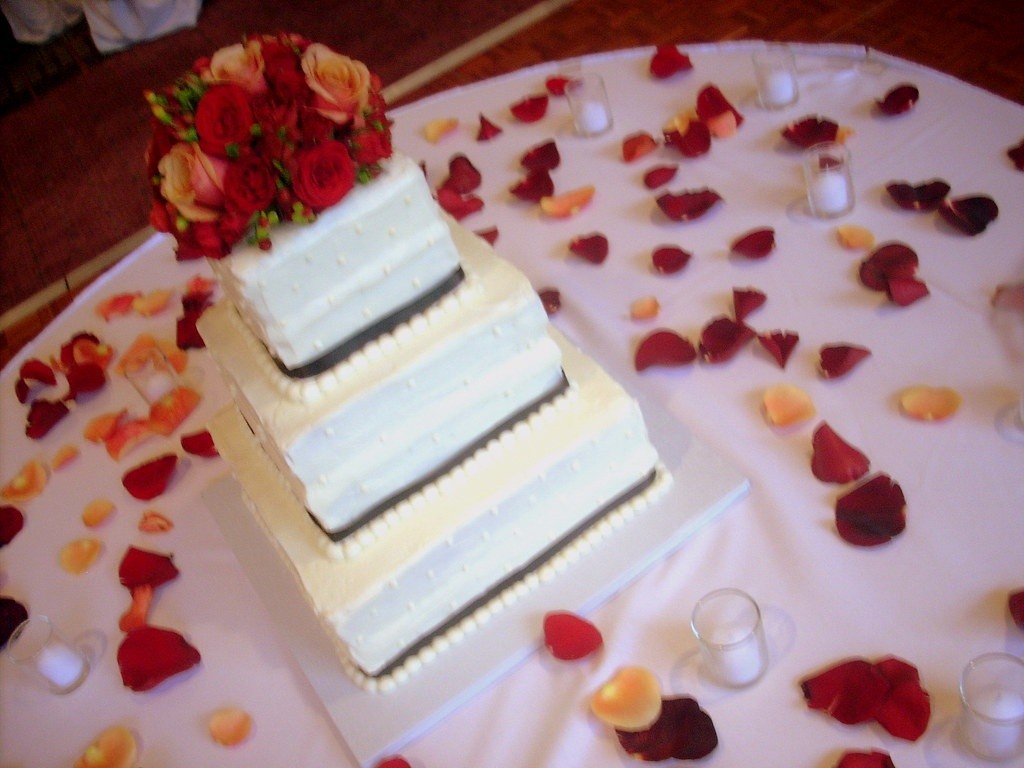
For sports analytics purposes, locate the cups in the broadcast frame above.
[122,348,182,406]
[801,142,855,219]
[6,614,90,695]
[691,588,769,688]
[565,73,612,138]
[750,42,801,111]
[958,652,1024,758]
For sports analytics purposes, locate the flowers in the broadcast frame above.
[144,26,396,266]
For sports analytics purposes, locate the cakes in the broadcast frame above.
[195,152,674,694]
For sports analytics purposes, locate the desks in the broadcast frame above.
[0,0,1024,768]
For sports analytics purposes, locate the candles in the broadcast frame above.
[760,68,795,106]
[576,98,608,134]
[144,368,175,402]
[968,683,1024,757]
[714,625,762,684]
[37,641,84,687]
[811,162,849,214]
